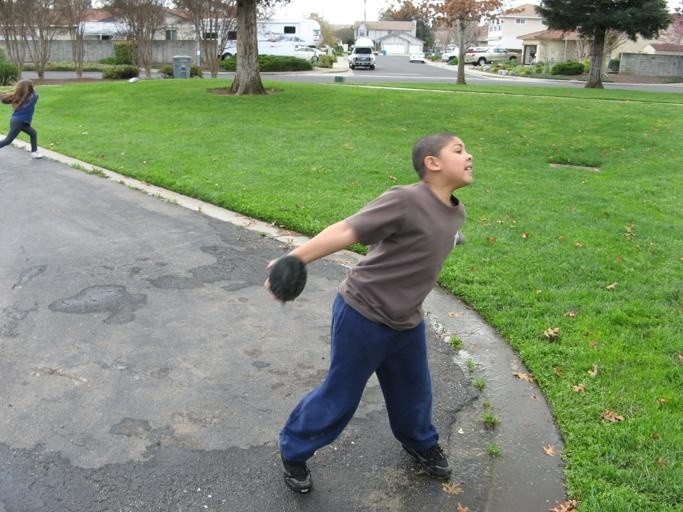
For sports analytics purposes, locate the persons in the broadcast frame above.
[264,130,477,495]
[0,78,45,161]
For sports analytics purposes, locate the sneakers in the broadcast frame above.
[281,452,313,493]
[402,444,452,480]
[32,152,42,158]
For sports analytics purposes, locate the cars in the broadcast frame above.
[465,46,519,67]
[291,47,325,63]
[266,33,309,50]
[409,52,425,63]
[441,47,459,63]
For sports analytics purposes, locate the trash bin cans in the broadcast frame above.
[383,51,386,55]
[172,55,192,79]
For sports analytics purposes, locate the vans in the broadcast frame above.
[349,46,375,70]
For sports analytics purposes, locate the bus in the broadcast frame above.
[193,17,322,62]
